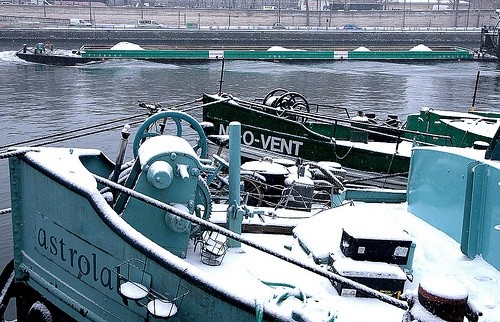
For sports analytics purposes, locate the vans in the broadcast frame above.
[138,19,163,29]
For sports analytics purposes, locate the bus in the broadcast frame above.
[330,2,384,11]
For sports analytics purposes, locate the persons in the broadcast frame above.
[358,111,364,117]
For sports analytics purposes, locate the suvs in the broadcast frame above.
[70,18,92,27]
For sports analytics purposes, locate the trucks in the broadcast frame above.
[432,5,450,10]
[263,5,278,10]
[70,19,92,28]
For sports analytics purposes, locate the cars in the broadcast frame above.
[392,8,411,10]
[272,23,287,29]
[122,1,259,10]
[343,23,362,29]
[186,23,199,29]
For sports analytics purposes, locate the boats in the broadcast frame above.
[15,42,104,67]
[201,91,500,194]
[1,145,500,322]
